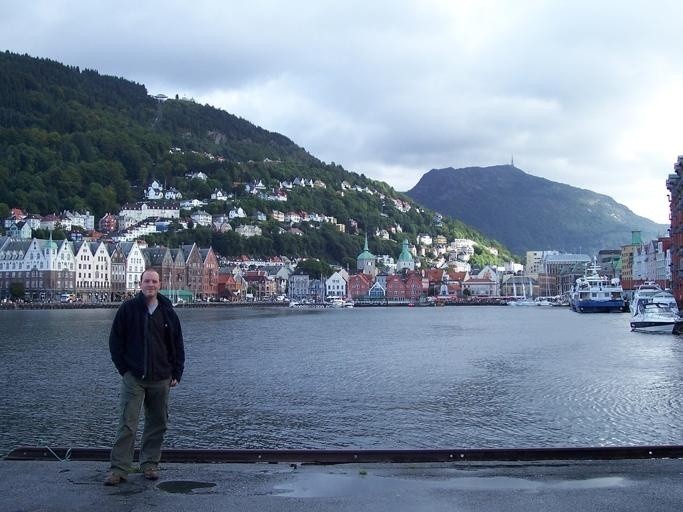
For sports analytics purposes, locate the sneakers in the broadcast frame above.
[103,471,129,486]
[143,461,160,480]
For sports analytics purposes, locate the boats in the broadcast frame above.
[504,257,683,336]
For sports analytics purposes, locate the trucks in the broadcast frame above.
[59,293,76,303]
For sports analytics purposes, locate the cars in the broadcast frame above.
[191,296,229,303]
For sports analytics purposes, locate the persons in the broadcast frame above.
[103,268,185,486]
